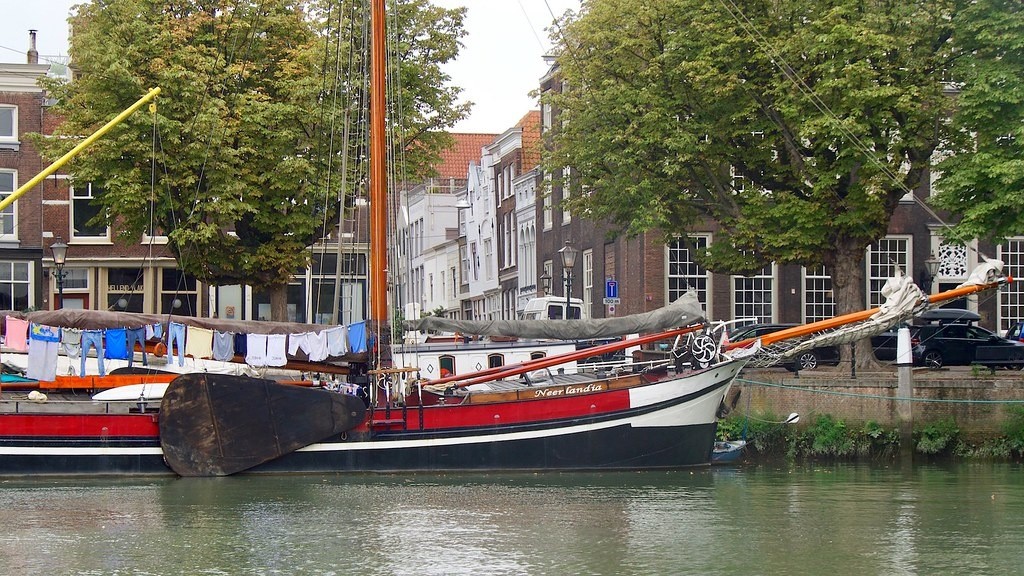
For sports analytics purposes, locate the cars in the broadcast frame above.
[724,305,1024,373]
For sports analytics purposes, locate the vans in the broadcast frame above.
[514,293,588,320]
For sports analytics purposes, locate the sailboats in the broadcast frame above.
[0,0,1014,488]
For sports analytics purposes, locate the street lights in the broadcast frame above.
[557,238,579,320]
[49,234,70,308]
